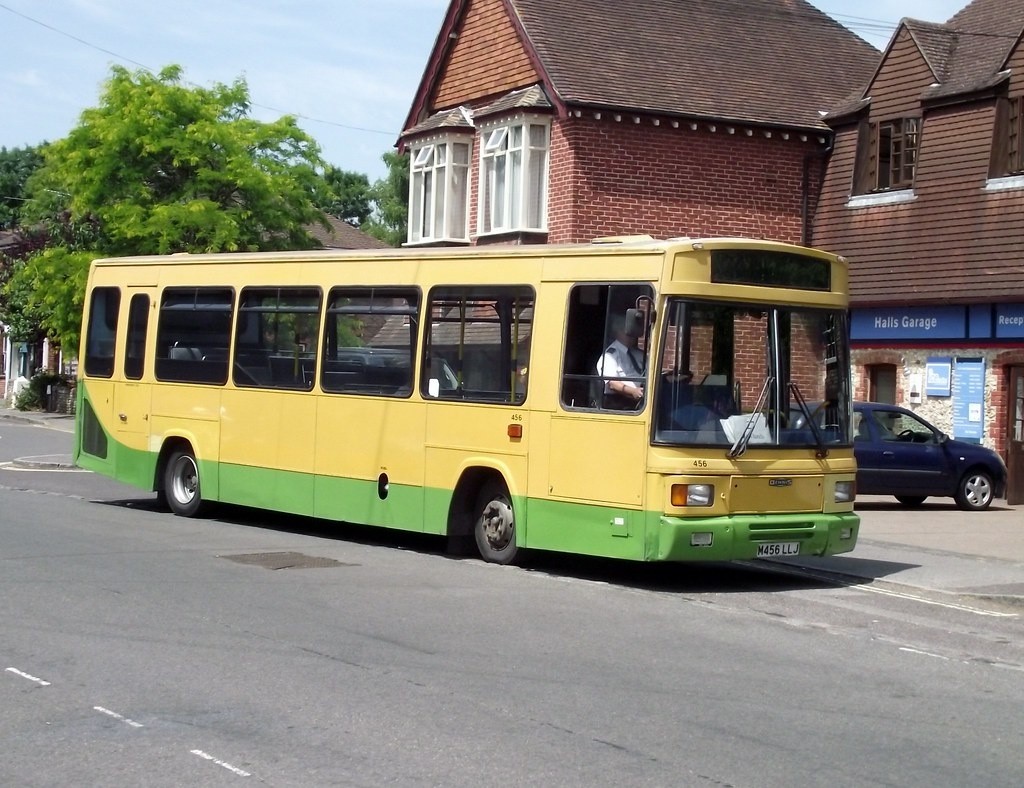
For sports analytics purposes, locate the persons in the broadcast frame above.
[596,303,670,412]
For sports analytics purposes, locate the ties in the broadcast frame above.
[627,350,642,375]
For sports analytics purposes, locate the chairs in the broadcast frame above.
[854,418,871,441]
[106,346,624,410]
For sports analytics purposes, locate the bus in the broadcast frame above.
[71,235,861,565]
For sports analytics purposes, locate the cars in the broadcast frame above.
[768,402,1007,512]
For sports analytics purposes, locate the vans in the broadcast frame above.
[308,347,466,398]
